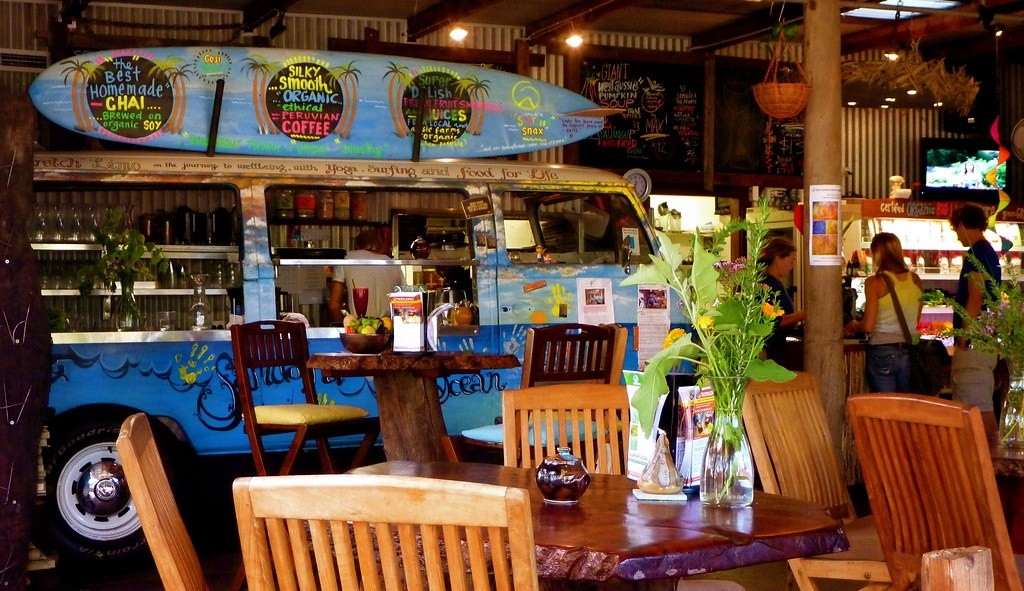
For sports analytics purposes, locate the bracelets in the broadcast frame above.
[852,321,858,331]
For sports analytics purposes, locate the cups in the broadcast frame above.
[352,287,369,318]
[455,307,474,326]
[413,270,438,290]
[158,311,176,331]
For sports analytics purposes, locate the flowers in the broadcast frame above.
[911,244,1024,372]
[618,194,797,449]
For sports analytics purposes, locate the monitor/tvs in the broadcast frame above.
[920,136,1016,203]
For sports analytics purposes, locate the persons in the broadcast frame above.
[329,230,408,325]
[840,232,924,393]
[747,237,805,371]
[950,205,1003,473]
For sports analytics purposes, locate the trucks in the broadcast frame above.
[16,46,699,573]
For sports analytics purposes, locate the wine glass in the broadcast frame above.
[30,201,135,244]
[38,251,241,332]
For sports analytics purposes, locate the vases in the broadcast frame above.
[698,376,756,508]
[997,356,1024,448]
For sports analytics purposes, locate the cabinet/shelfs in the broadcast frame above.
[28,215,479,297]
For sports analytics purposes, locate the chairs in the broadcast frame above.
[115,318,1024,591]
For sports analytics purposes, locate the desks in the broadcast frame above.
[348,462,851,591]
[305,345,522,462]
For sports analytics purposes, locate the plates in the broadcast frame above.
[275,247,347,259]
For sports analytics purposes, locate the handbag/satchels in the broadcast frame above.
[910,338,952,396]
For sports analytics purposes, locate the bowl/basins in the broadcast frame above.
[339,332,390,354]
[893,189,911,199]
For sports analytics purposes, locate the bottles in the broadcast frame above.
[273,187,368,221]
[636,429,685,493]
[536,446,591,505]
[410,235,431,261]
[657,201,681,233]
